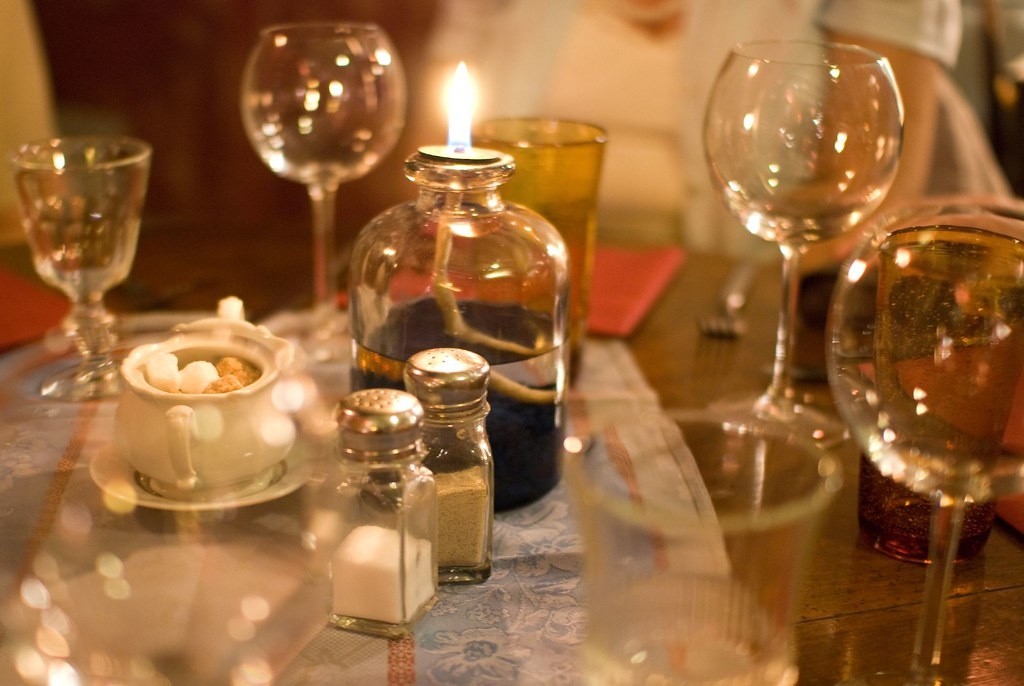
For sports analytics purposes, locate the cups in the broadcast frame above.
[851,450,998,567]
[470,117,607,386]
[113,325,297,504]
[567,397,838,686]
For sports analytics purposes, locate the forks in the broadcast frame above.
[695,264,759,338]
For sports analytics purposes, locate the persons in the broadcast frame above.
[380,0,1012,241]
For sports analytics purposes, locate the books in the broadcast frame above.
[0,261,74,354]
[333,244,688,338]
[856,346,1024,537]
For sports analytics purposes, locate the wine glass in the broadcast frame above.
[704,37,903,449]
[8,132,155,400]
[239,21,408,349]
[827,224,1024,685]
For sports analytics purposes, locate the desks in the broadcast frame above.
[0,249,1024,683]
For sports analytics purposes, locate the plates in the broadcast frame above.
[89,435,318,512]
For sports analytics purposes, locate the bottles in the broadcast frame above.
[343,140,567,512]
[300,381,443,642]
[402,350,493,587]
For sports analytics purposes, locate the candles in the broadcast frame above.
[346,57,568,509]
